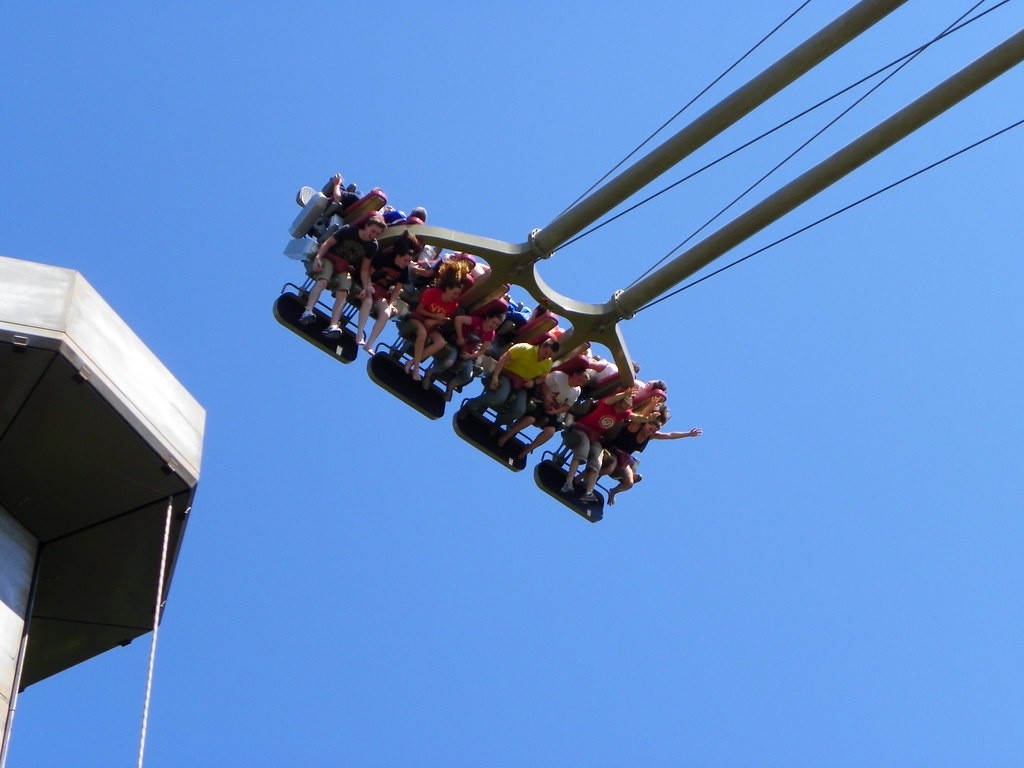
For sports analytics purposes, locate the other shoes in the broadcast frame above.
[405,361,412,373]
[445,386,453,401]
[424,370,436,389]
[581,492,599,506]
[562,481,574,492]
[518,444,532,461]
[490,420,501,435]
[363,343,375,355]
[498,433,513,447]
[355,333,367,345]
[413,370,422,381]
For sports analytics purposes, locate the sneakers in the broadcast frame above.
[322,325,342,338]
[298,306,316,325]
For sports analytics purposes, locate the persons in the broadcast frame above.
[459,300,640,460]
[297,172,426,357]
[402,246,509,403]
[559,380,702,507]
